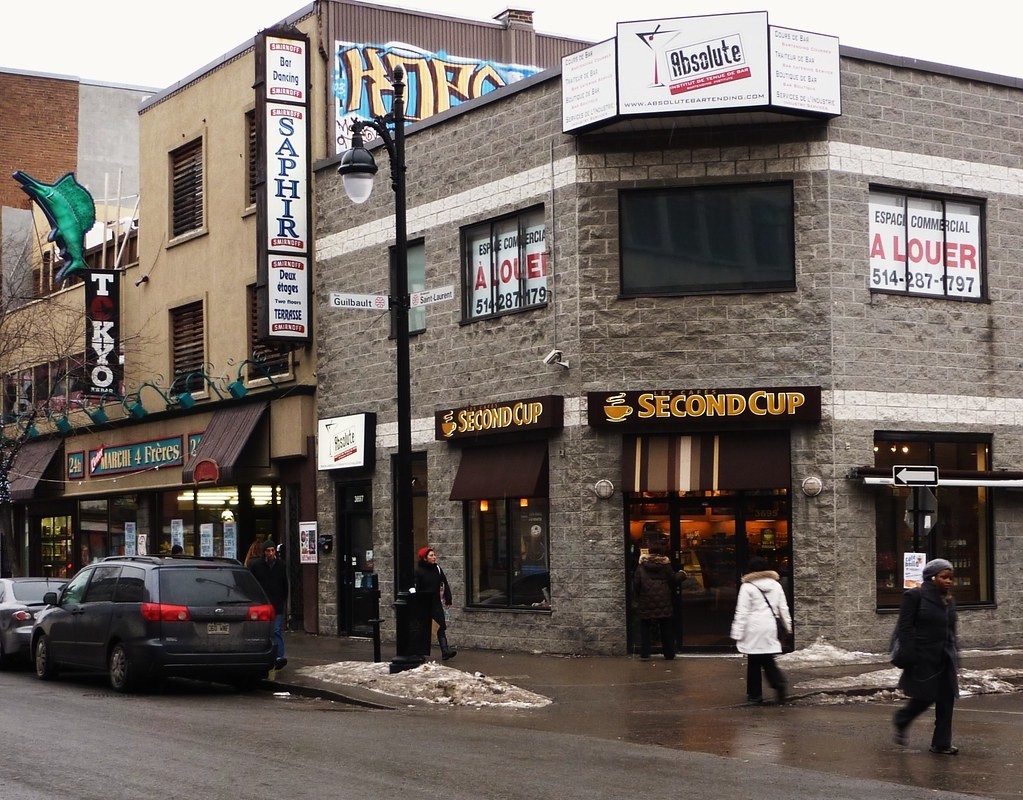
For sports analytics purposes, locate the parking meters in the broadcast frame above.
[29,552,276,691]
[366,574,383,663]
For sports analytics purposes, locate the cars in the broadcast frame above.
[0,577,72,669]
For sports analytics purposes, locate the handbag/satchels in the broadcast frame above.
[889,635,913,670]
[776,618,794,653]
[436,565,445,601]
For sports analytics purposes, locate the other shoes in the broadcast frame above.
[664,653,675,660]
[775,682,787,705]
[275,658,287,671]
[639,652,650,660]
[746,694,764,702]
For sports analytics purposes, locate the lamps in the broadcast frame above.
[802,477,823,497]
[595,479,614,499]
[221,499,235,526]
[0,348,279,445]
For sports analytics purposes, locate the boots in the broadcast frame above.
[438,636,457,660]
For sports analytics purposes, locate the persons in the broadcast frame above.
[893,559,960,754]
[244,541,264,569]
[633,545,687,660]
[414,546,457,660]
[252,540,289,670]
[731,558,792,702]
[171,545,185,555]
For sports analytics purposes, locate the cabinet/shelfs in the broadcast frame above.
[636,546,710,636]
[943,545,973,591]
[494,499,549,571]
[41,543,72,557]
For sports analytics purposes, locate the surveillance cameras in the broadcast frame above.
[543,349,562,364]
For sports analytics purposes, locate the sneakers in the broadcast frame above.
[891,712,911,745]
[929,743,960,755]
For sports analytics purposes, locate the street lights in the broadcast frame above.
[336,62,427,677]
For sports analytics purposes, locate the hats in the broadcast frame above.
[171,545,183,555]
[740,559,765,576]
[262,541,276,554]
[417,547,433,560]
[923,559,954,581]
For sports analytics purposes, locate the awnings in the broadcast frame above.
[621,431,791,491]
[182,398,269,484]
[7,437,63,500]
[449,438,551,502]
